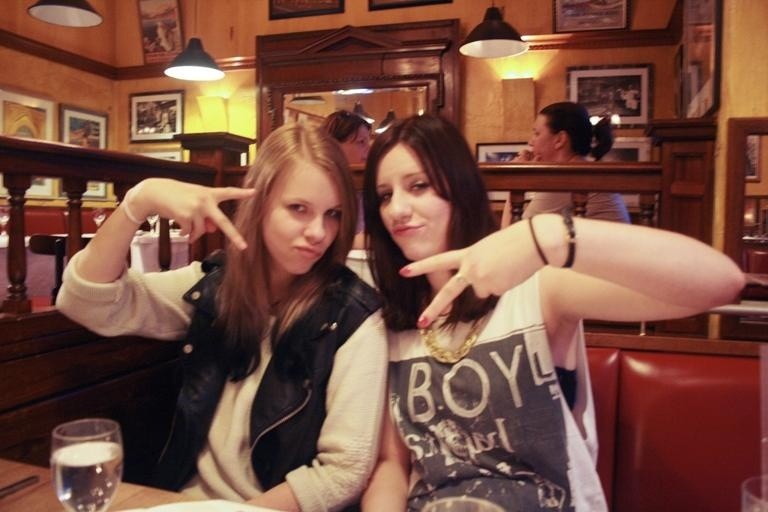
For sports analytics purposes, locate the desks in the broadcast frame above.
[0,228,189,296]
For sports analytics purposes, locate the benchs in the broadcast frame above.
[578,334,768,511]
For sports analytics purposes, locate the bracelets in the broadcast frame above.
[528,215,550,266]
[122,187,147,226]
[555,212,578,268]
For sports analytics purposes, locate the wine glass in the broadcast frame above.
[90,205,107,228]
[144,214,175,237]
[0,205,12,236]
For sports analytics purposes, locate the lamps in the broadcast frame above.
[163,1,226,83]
[27,0,105,29]
[458,0,529,60]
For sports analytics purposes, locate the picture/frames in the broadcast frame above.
[0,84,109,202]
[565,63,654,130]
[551,1,633,35]
[472,137,652,209]
[128,90,184,143]
[135,0,184,68]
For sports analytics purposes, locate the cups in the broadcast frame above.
[49,417,124,511]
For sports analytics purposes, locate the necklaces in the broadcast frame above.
[419,308,490,364]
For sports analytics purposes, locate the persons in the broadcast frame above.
[498,102,634,230]
[56,121,387,510]
[361,112,749,510]
[321,109,380,252]
[620,83,640,111]
